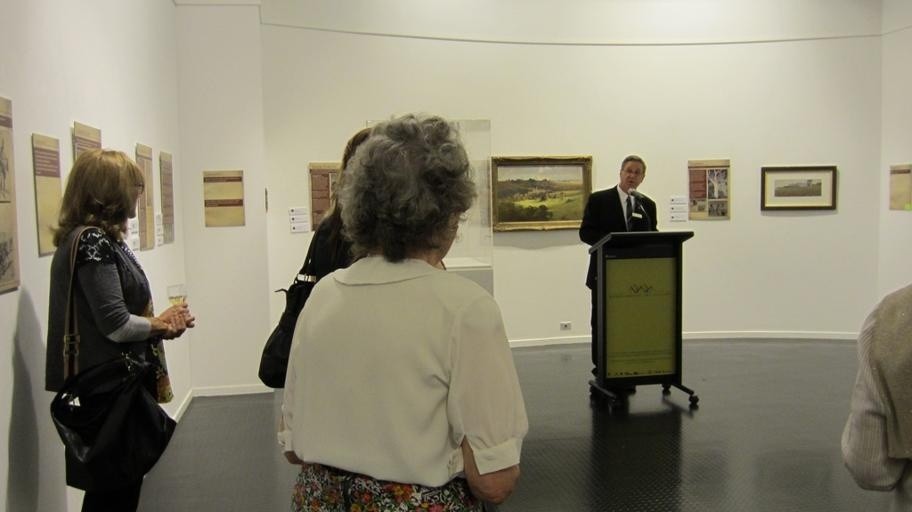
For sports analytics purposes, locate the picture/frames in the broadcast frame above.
[761,166,837,210]
[491,155,591,233]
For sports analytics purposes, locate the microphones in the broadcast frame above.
[626,187,645,202]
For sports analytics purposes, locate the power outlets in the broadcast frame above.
[560,321,571,331]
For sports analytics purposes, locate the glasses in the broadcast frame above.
[134,183,145,195]
[621,168,643,176]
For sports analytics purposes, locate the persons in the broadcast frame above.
[580,155,657,396]
[282,113,528,512]
[258,129,447,388]
[841,283,912,512]
[45,149,195,512]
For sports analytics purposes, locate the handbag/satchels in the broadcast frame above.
[259,275,315,390]
[47,353,177,494]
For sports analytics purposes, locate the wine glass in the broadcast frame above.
[167,284,186,306]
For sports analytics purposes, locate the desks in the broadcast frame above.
[442,257,494,299]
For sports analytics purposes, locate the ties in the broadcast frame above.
[625,193,635,228]
